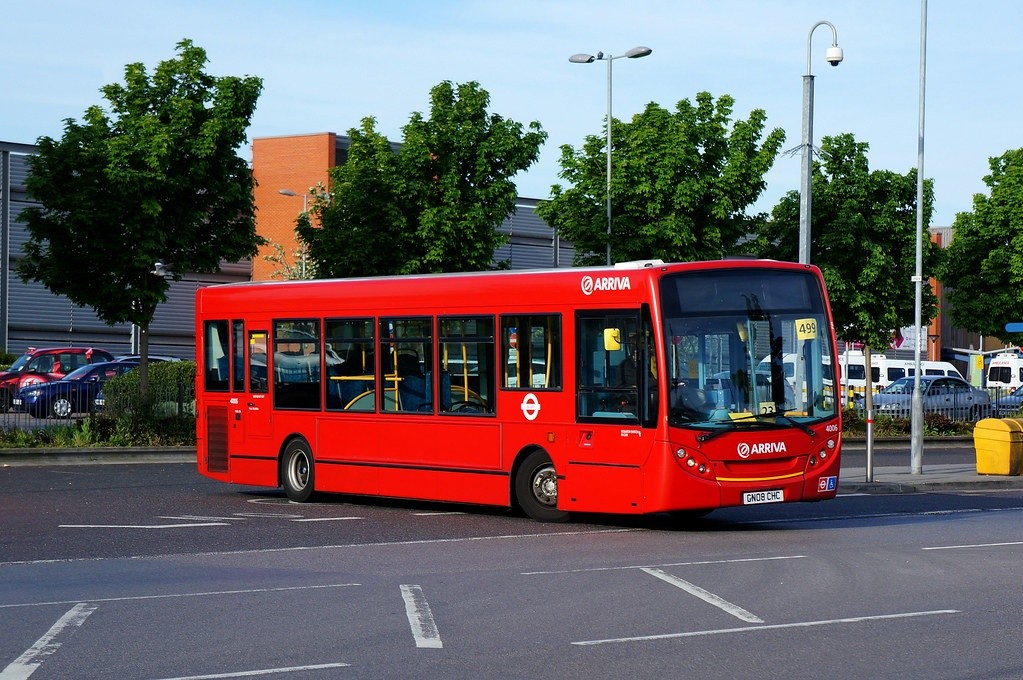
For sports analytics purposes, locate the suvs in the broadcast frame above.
[114,354,185,365]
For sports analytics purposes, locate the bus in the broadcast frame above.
[195,258,843,524]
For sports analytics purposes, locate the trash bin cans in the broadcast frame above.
[973,418,1023,477]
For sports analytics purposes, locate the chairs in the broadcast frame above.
[217,350,742,425]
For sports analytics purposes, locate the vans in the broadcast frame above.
[757,353,866,409]
[987,352,1023,391]
[869,357,966,395]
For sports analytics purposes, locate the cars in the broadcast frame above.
[0,346,116,412]
[702,370,798,413]
[12,360,140,420]
[992,384,1023,419]
[856,376,991,422]
[458,359,552,391]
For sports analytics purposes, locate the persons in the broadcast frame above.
[614,335,686,413]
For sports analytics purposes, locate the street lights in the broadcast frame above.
[276,187,335,278]
[569,46,652,264]
